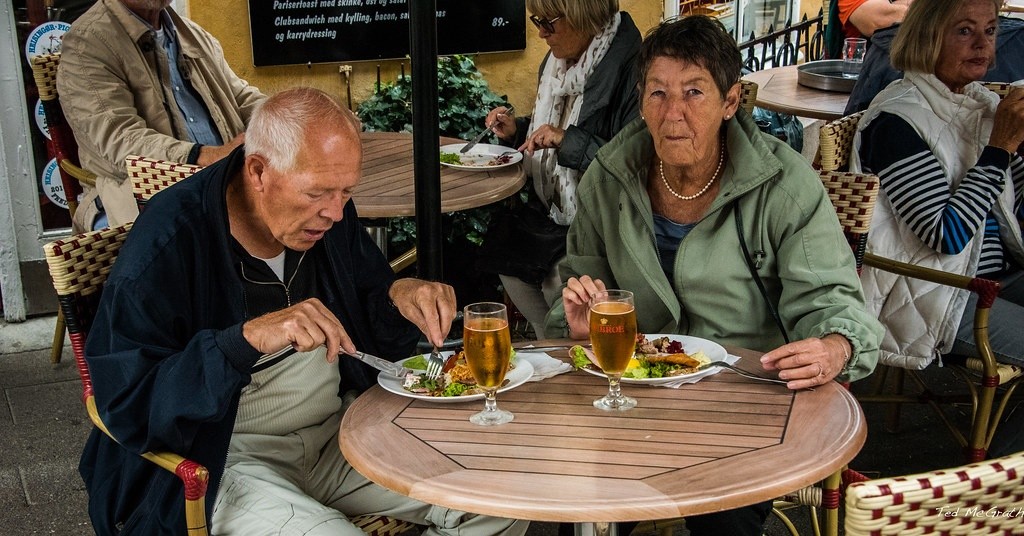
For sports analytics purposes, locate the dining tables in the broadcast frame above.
[336,335,870,536]
[351,130,528,275]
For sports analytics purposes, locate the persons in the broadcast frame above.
[81,84,531,536]
[481,0,648,341]
[541,14,877,536]
[823,0,1024,365]
[54,0,268,225]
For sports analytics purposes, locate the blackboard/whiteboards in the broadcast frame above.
[247,0,527,68]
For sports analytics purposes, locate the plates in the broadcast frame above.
[572,333,728,385]
[439,143,524,172]
[377,351,534,403]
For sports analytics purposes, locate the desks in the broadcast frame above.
[739,65,851,171]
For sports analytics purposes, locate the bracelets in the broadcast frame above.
[838,338,849,369]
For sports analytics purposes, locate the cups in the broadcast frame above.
[1010,79,1024,91]
[842,37,867,78]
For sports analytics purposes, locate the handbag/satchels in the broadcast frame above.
[479,205,548,278]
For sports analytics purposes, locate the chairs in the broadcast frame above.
[38,218,427,536]
[772,164,881,536]
[820,105,1024,465]
[843,450,1024,536]
[124,154,209,217]
[30,51,99,220]
[977,79,1012,103]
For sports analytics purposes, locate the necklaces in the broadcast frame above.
[660,131,726,200]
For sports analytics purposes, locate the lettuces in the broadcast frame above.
[569,344,684,380]
[402,347,517,398]
[441,151,465,166]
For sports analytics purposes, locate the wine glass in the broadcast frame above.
[589,289,638,412]
[463,302,514,426]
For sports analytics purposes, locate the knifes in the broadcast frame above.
[513,346,591,354]
[339,345,410,378]
[460,107,513,153]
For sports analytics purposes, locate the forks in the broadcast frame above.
[501,150,521,156]
[698,360,815,391]
[424,344,444,381]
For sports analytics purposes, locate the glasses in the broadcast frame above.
[529,14,561,34]
